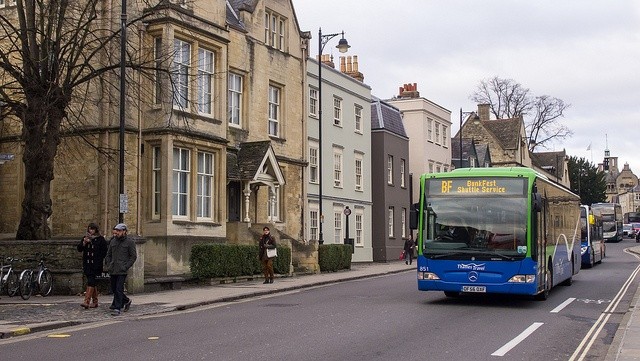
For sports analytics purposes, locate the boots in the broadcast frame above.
[80,287,94,309]
[90,287,98,308]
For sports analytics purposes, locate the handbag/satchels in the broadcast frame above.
[400,251,406,260]
[266,239,277,258]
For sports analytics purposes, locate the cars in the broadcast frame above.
[635,224,640,237]
[623,223,635,239]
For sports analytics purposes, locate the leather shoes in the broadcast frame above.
[270,278,274,284]
[111,310,120,316]
[124,298,132,311]
[263,280,269,283]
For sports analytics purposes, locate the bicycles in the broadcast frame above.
[0,256,19,297]
[19,252,52,299]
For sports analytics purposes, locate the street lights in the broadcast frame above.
[316,26,351,245]
[519,134,536,166]
[460,107,481,167]
[556,154,569,186]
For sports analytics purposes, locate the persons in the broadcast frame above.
[259,227,276,284]
[404,234,415,265]
[77,223,106,308]
[106,224,137,316]
[435,217,468,242]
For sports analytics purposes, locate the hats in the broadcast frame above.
[115,224,127,230]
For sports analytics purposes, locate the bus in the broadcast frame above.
[592,203,624,242]
[417,167,582,301]
[624,212,640,224]
[580,204,606,267]
